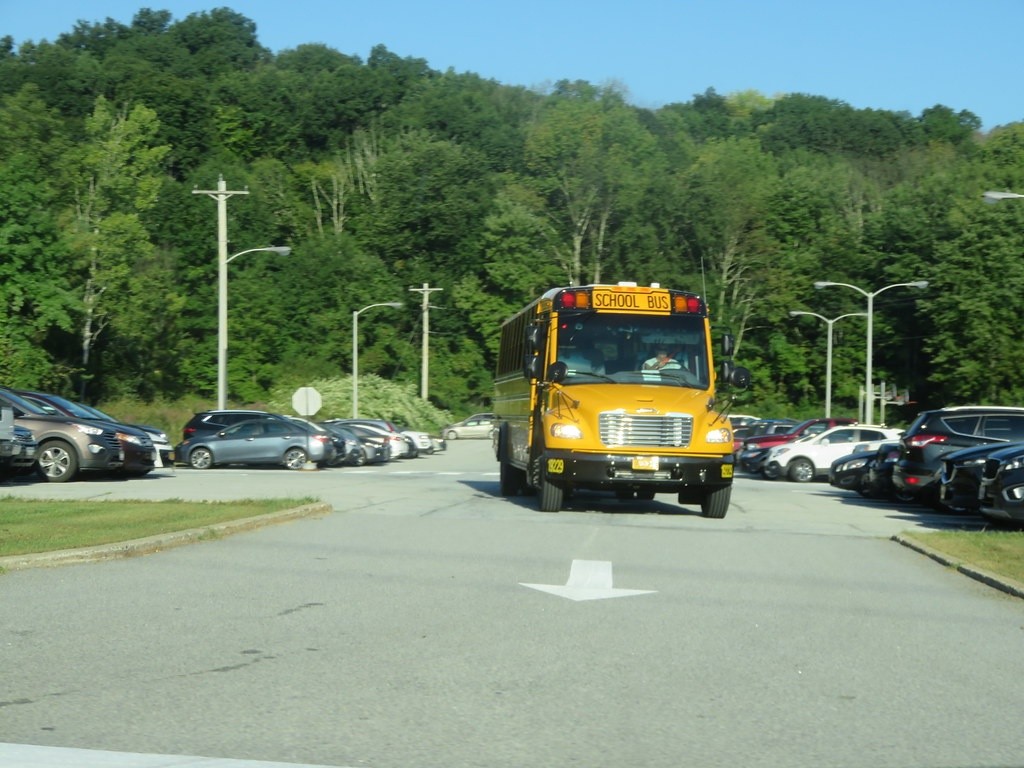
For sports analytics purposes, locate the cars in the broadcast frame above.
[728,412,908,503]
[173,418,331,469]
[938,440,1023,515]
[183,408,447,468]
[442,418,493,441]
[0,421,39,469]
[978,442,1024,524]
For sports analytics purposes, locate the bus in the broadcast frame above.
[490,281,752,519]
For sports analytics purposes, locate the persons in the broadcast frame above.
[641,343,681,370]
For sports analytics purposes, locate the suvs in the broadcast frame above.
[0,385,125,483]
[15,390,156,471]
[892,405,1024,514]
[454,412,493,427]
[73,400,176,477]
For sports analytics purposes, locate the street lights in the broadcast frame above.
[215,246,293,411]
[789,310,869,418]
[352,302,403,420]
[812,279,930,425]
